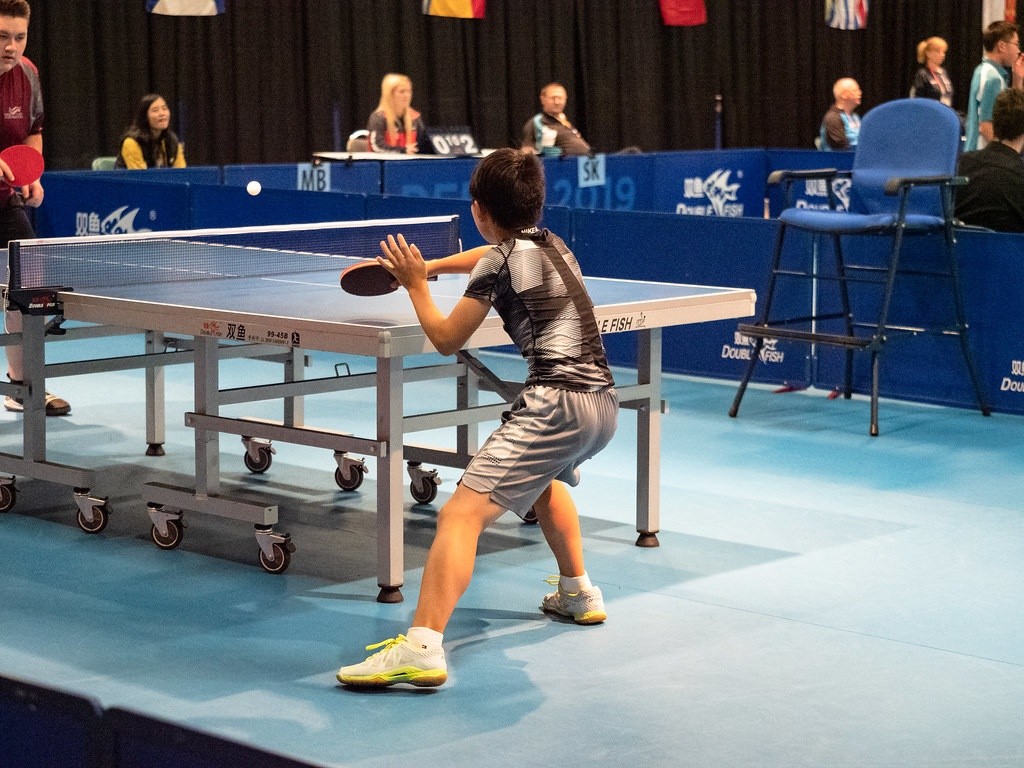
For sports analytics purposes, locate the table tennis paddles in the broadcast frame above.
[0,145,45,187]
[338,261,437,297]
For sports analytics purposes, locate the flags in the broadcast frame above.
[982,0,1017,32]
[146,0,226,16]
[423,0,486,18]
[824,0,868,30]
[659,0,707,27]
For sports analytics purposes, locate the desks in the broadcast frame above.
[0,234,755,603]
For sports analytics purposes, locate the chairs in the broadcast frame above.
[729,97,992,438]
[92,157,117,171]
[346,130,371,152]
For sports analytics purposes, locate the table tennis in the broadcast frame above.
[247,181,262,196]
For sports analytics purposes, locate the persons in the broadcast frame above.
[909,36,953,107]
[821,77,862,152]
[113,94,187,170]
[0,0,71,416]
[954,87,1024,234]
[522,83,593,155]
[368,73,434,154]
[336,148,620,687]
[964,22,1024,152]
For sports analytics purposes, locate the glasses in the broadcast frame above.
[1002,40,1020,49]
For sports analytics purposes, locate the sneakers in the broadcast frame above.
[3,373,71,416]
[541,574,608,624]
[336,633,448,688]
